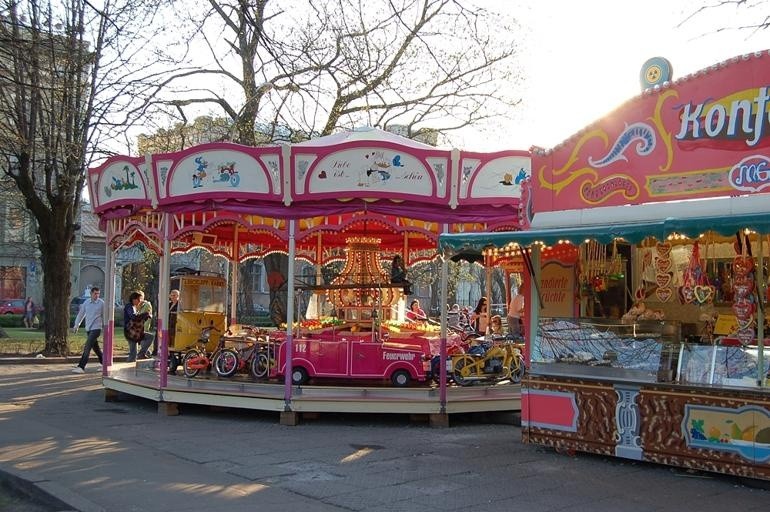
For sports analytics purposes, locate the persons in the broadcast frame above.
[390,253,415,295]
[122,293,157,363]
[69,286,106,374]
[168,289,178,347]
[492,313,505,335]
[470,296,490,324]
[135,290,153,357]
[22,295,35,329]
[437,302,476,330]
[151,294,159,357]
[506,283,525,335]
[406,301,426,325]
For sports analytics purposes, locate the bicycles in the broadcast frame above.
[180,325,272,378]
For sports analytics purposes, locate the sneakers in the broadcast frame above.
[138,353,149,358]
[97,367,103,371]
[72,367,84,374]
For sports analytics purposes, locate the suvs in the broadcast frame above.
[70,296,123,313]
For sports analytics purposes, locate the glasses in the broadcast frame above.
[483,304,486,306]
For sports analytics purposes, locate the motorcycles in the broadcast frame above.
[451,333,525,386]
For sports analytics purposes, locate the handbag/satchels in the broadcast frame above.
[124,318,145,342]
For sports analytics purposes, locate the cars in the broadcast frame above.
[0,298,45,317]
[272,321,433,385]
